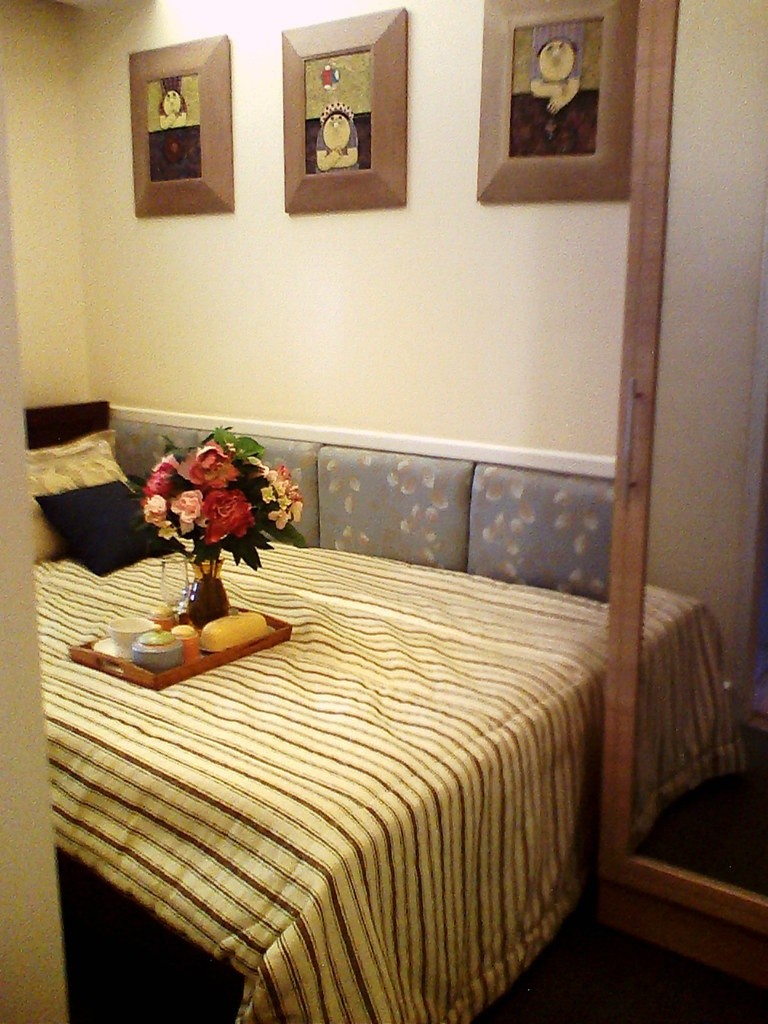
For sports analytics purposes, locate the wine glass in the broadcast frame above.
[161,556,189,626]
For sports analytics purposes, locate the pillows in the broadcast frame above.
[28,429,138,571]
[31,479,185,576]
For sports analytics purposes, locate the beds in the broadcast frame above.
[32,416,617,1024]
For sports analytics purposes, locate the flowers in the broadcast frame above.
[139,427,306,573]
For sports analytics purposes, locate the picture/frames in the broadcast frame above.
[477,1,638,202]
[129,35,235,217]
[281,9,408,212]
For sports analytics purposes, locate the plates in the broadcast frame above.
[94,638,132,662]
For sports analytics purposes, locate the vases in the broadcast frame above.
[186,558,229,632]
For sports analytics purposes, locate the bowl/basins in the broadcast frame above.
[108,619,153,652]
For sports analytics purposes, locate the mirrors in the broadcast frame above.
[597,0,768,986]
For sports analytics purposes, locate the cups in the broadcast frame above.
[176,632,199,663]
[148,615,176,630]
